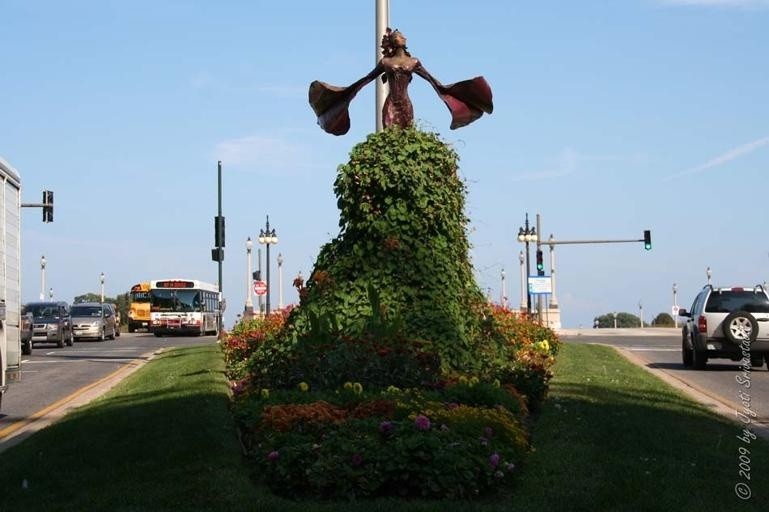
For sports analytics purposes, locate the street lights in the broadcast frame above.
[100,272,105,304]
[40,256,53,302]
[594,267,712,328]
[245,213,284,317]
[500,212,558,322]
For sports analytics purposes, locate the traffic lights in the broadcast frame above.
[644,230,652,249]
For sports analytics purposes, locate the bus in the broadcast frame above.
[128,278,224,336]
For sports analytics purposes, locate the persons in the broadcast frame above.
[309,27,495,136]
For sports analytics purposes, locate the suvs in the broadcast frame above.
[679,284,769,371]
[21,302,120,353]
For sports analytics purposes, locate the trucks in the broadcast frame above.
[0,158,21,413]
